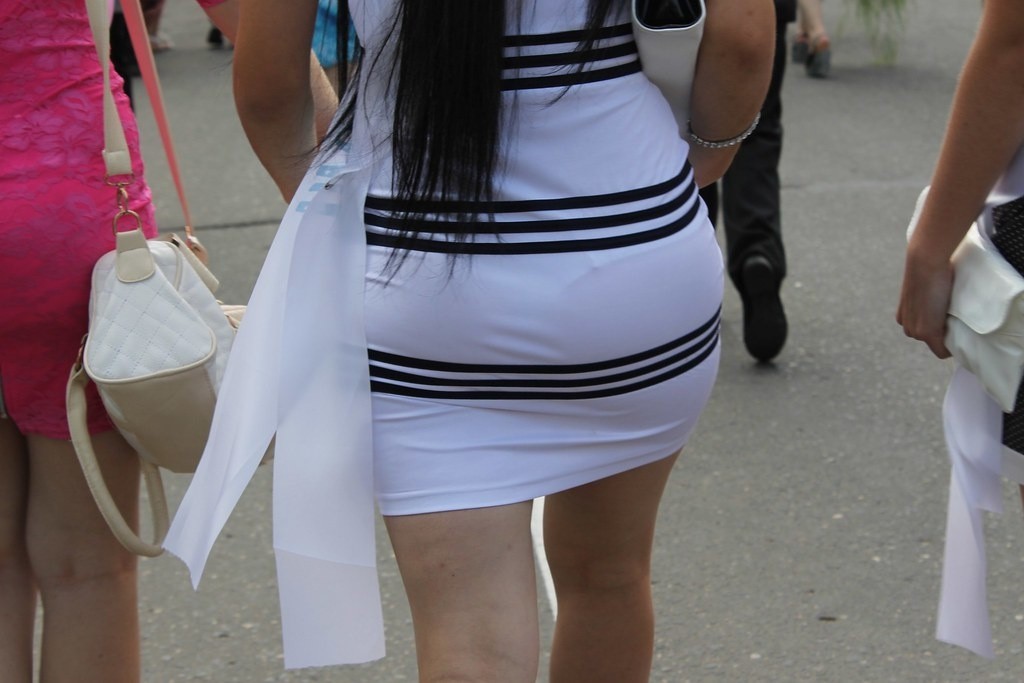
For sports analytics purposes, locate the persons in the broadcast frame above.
[109,0,830,360]
[1,0,159,683]
[896,0,1024,454]
[233,0,777,683]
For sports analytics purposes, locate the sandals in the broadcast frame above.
[149,34,175,53]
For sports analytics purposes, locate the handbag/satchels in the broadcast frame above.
[66,227,275,557]
[905,186,1024,414]
[630,0,706,139]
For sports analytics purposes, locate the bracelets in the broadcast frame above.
[688,112,761,148]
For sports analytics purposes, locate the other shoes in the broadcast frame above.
[207,27,233,49]
[792,33,831,78]
[741,256,788,362]
